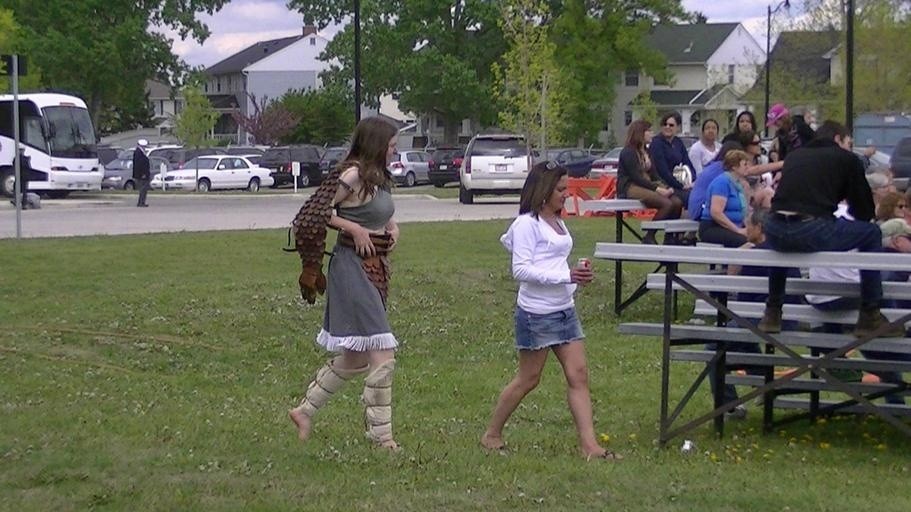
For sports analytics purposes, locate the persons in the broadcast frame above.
[289,115,404,452]
[477,158,625,463]
[131,136,154,209]
[617,111,696,245]
[687,102,911,420]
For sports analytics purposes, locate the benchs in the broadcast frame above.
[578,193,910,453]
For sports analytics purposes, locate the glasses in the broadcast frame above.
[545,158,560,171]
[663,121,674,127]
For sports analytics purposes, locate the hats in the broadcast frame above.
[766,104,788,125]
[138,139,149,146]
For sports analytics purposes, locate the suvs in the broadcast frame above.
[458,130,542,205]
[888,137,911,190]
[427,144,466,188]
[256,143,341,186]
[318,146,348,178]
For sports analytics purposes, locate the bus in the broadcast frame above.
[0,92,107,200]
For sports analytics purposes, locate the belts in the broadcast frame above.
[770,212,805,223]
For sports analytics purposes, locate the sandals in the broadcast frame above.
[480,432,510,452]
[588,449,622,460]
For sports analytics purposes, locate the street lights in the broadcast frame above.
[764,0,792,136]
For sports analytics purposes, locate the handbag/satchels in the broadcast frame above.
[673,164,695,188]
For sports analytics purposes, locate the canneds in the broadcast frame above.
[577,258,591,272]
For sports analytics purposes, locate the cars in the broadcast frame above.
[590,146,622,170]
[96,143,266,171]
[677,135,698,152]
[547,145,599,179]
[387,149,432,188]
[852,145,891,176]
[147,154,277,193]
[101,155,175,192]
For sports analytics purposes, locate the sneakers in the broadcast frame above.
[855,313,904,337]
[759,310,781,332]
[754,393,766,406]
[727,405,747,418]
[642,232,698,245]
